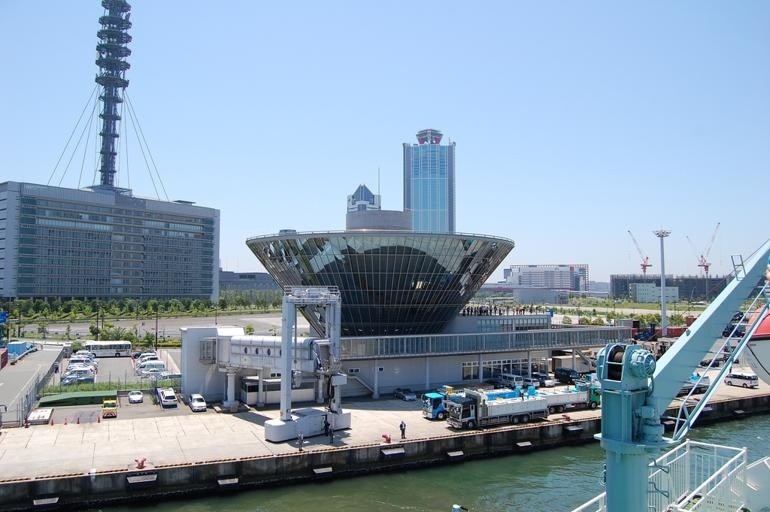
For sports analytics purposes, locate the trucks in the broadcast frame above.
[422,382,602,434]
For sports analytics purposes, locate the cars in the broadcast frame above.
[392,385,417,402]
[61,349,168,386]
[102,399,118,419]
[188,393,207,413]
[127,390,144,403]
[700,311,749,370]
[158,388,179,409]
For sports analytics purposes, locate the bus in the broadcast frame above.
[82,340,133,358]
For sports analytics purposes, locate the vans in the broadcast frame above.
[496,367,582,389]
[723,371,760,388]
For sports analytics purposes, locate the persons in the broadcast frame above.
[329,426,335,444]
[400,421,407,439]
[324,420,330,437]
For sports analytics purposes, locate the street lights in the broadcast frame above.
[0,404,9,414]
[450,504,469,512]
